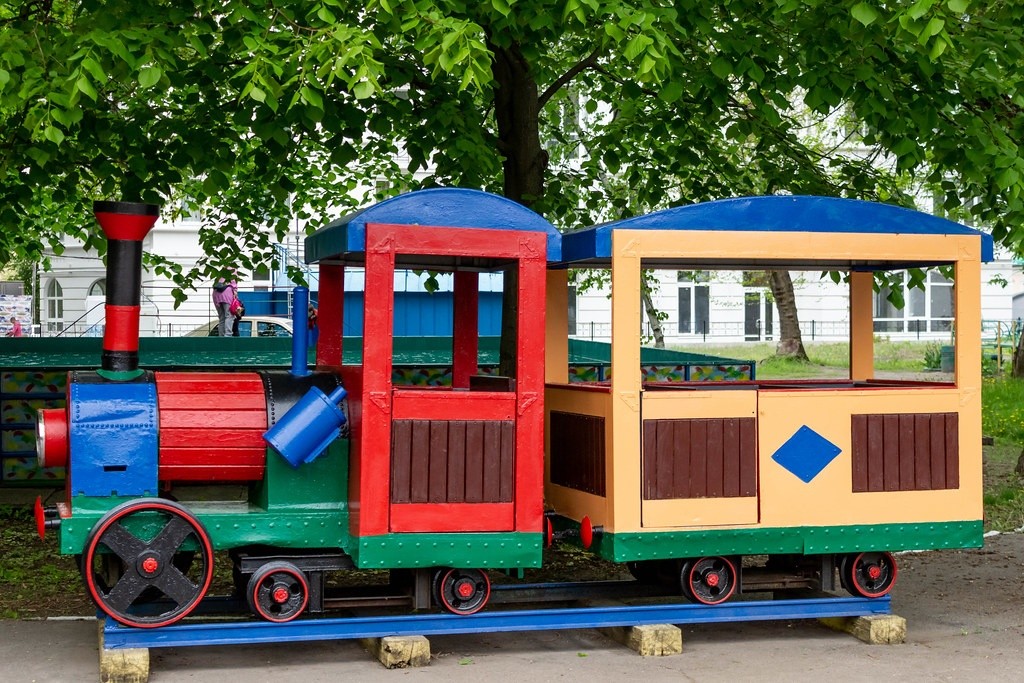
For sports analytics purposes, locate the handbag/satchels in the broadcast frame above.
[213,278,229,292]
[230,299,245,320]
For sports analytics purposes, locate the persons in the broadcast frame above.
[233,299,244,336]
[212,277,237,336]
[7,317,21,337]
[308,305,318,324]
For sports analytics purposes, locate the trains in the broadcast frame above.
[30,186,996,628]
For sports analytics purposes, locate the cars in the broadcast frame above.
[182,316,293,336]
